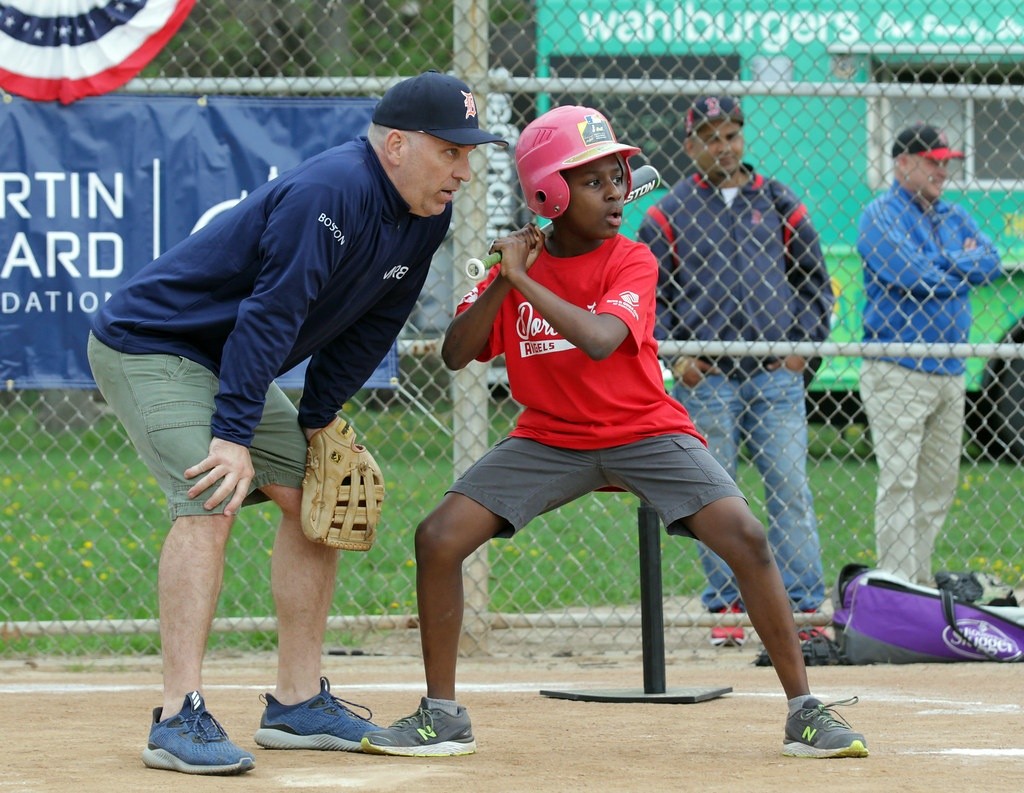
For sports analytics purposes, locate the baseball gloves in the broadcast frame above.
[296,415,388,553]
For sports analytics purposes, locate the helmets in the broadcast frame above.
[515,105,641,219]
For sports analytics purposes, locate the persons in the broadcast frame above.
[859,124,1000,587]
[88,69,508,775]
[637,92,833,647]
[360,104,870,756]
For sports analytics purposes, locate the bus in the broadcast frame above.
[536,1,1023,461]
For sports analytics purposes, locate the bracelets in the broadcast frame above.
[672,357,689,377]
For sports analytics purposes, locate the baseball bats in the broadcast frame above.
[462,162,662,281]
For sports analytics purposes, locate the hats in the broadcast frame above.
[371,69,509,148]
[685,94,744,137]
[893,121,965,162]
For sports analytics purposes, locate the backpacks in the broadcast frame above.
[830,569,1024,666]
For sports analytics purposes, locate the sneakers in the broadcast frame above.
[781,696,869,759]
[141,691,256,776]
[710,600,744,647]
[361,697,476,757]
[797,609,831,645]
[254,677,387,753]
